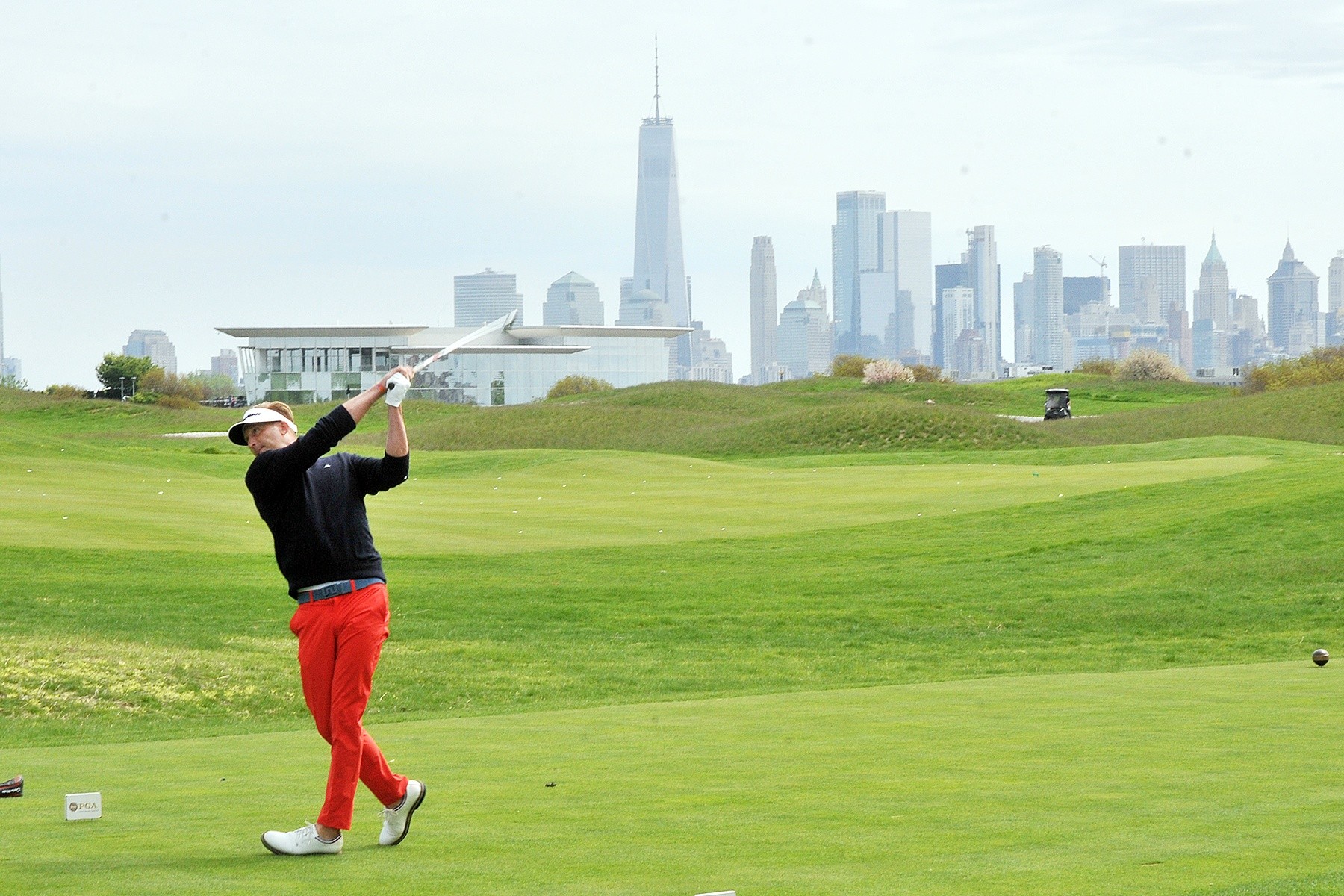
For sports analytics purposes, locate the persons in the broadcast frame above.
[228,365,427,856]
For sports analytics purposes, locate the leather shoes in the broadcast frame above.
[260,821,343,855]
[378,779,426,846]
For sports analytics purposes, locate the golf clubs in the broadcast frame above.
[388,308,519,390]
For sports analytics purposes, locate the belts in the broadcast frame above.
[298,577,384,605]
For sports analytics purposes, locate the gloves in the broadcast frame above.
[384,372,411,407]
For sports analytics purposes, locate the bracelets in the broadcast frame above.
[376,382,387,394]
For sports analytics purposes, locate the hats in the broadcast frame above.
[228,408,297,446]
[233,396,235,397]
